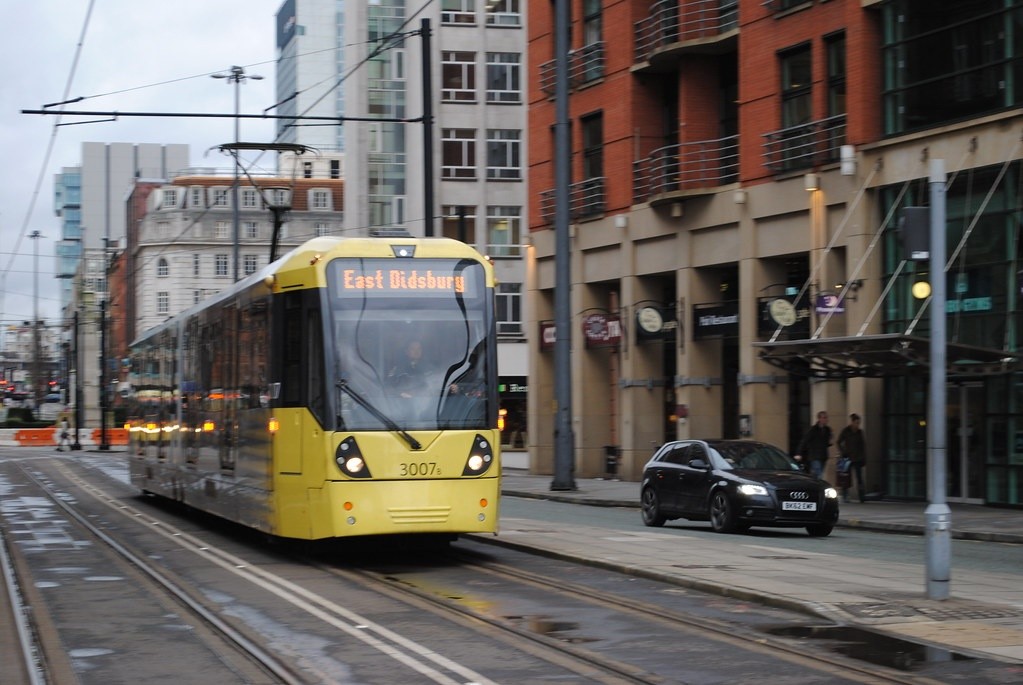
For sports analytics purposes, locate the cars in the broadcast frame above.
[641,438,840,537]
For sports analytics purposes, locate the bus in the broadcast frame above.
[119,142,506,552]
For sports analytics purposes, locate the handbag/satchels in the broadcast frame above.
[835,456,852,472]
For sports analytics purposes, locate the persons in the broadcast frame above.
[837,413,870,503]
[387,340,439,389]
[793,411,833,479]
[57,416,75,452]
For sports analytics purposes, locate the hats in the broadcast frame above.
[850,413,860,421]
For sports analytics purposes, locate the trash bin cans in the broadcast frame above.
[604,445,618,473]
